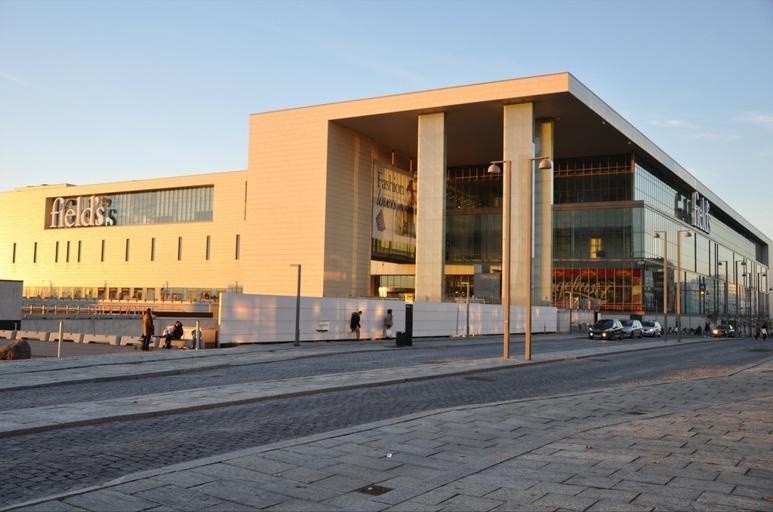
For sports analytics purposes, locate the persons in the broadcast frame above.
[759,325,767,342]
[140,307,154,351]
[349,310,362,341]
[380,308,394,337]
[160,319,183,350]
[703,319,714,338]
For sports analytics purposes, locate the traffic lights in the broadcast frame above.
[700,284,705,296]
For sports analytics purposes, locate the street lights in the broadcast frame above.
[524,153,553,361]
[489,160,513,362]
[674,226,693,341]
[289,263,304,346]
[651,228,669,336]
[717,258,772,340]
[460,282,470,337]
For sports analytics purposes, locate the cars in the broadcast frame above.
[586,318,662,341]
[712,324,735,338]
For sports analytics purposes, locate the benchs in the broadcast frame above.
[0,326,201,359]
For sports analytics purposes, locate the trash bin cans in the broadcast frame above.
[396,332,407,347]
[192,330,201,349]
